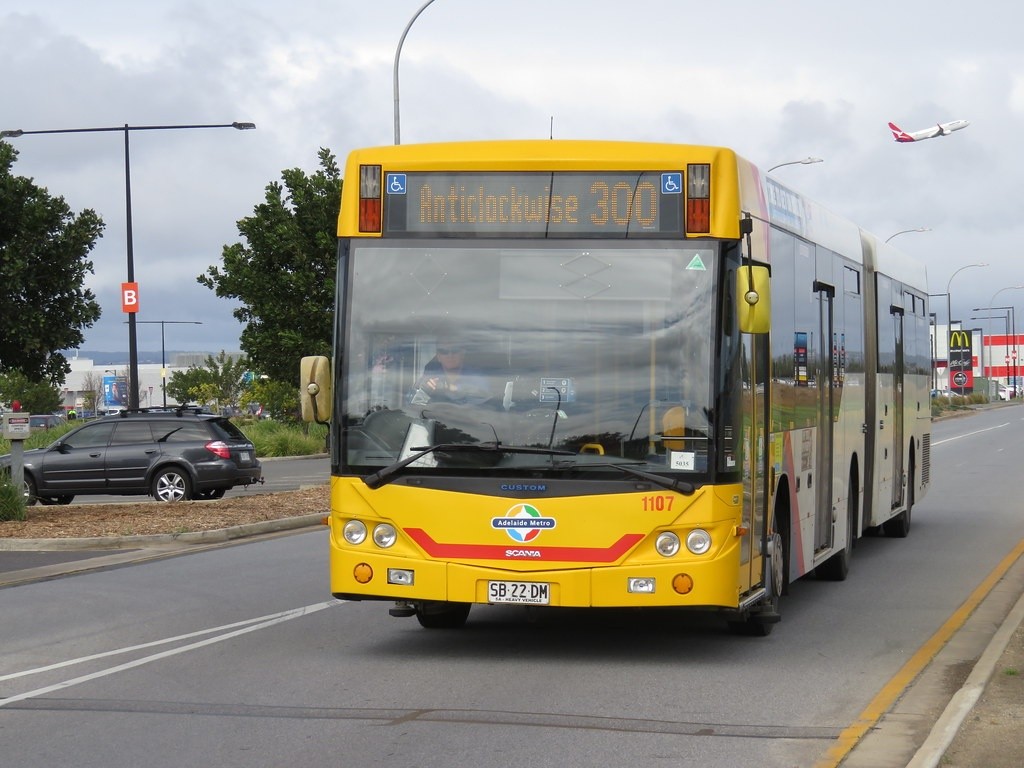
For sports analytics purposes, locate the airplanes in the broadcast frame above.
[888,118,972,144]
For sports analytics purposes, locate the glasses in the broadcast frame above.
[439,347,462,355]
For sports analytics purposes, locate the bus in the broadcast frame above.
[300,136,935,636]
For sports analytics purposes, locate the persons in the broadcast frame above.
[942,386,949,397]
[255,403,266,419]
[410,331,496,411]
[67,407,77,420]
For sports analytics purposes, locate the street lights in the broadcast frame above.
[765,155,825,174]
[970,306,1017,399]
[970,316,1011,402]
[122,321,203,407]
[105,370,116,375]
[0,121,258,408]
[946,262,989,406]
[883,229,930,247]
[988,285,1023,402]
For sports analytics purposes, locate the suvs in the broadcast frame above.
[0,409,262,501]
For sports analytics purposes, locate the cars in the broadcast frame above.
[103,409,123,417]
[931,379,1023,399]
[245,405,271,418]
[0,415,73,433]
